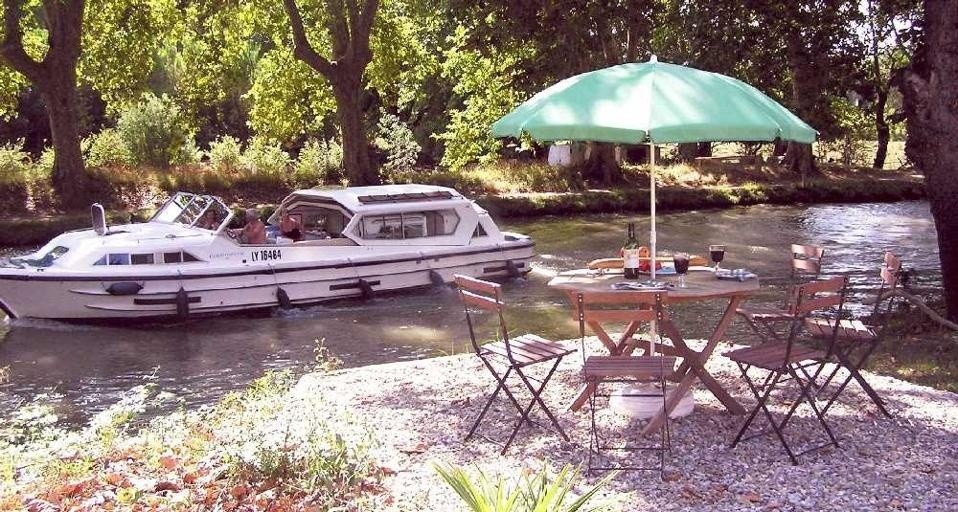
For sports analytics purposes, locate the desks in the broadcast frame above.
[549,262,761,439]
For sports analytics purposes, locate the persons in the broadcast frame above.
[202,204,302,243]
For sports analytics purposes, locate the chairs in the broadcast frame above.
[735,243,824,378]
[571,289,676,483]
[449,273,575,458]
[806,252,902,420]
[724,275,848,464]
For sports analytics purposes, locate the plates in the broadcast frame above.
[624,281,666,290]
[716,269,757,280]
[637,266,676,275]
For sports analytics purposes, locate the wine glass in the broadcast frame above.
[708,244,725,273]
[672,249,692,289]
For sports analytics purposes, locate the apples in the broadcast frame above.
[620,246,624,257]
[639,246,649,257]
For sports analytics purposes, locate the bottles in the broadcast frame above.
[621,221,641,279]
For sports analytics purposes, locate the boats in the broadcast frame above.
[0,186,539,331]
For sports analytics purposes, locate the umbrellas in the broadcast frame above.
[490,54,818,386]
